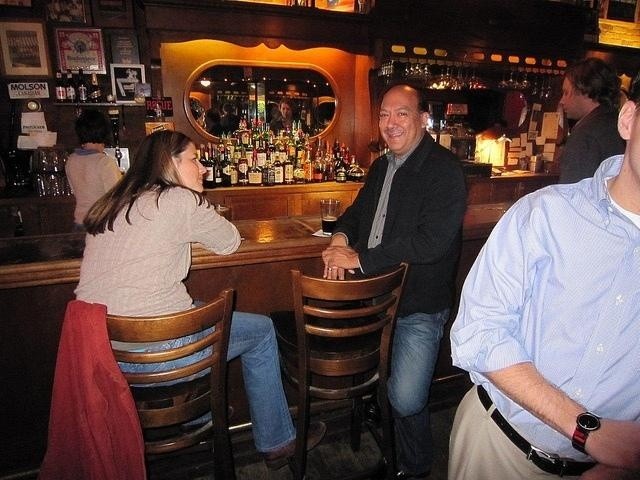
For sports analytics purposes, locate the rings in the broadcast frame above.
[327,267,333,271]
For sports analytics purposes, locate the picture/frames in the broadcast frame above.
[0,19,52,81]
[110,63,145,104]
[54,26,108,76]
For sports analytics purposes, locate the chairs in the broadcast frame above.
[69,288,235,479]
[270,262,410,479]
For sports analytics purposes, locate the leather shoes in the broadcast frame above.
[384,463,431,479]
[262,421,327,470]
[365,402,378,425]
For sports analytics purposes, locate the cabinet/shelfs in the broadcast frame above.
[369,37,570,158]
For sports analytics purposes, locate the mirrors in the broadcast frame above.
[183,59,342,143]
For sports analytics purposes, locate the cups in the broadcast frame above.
[320,199,341,235]
[214,204,230,221]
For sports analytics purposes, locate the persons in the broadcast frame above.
[220,102,241,134]
[73,130,327,469]
[66,109,123,230]
[321,81,466,480]
[205,109,226,137]
[447,76,640,480]
[557,59,625,185]
[272,96,314,140]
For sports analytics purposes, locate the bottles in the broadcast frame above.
[66,69,76,103]
[377,63,391,85]
[234,119,304,138]
[38,155,50,197]
[62,150,75,196]
[90,73,101,103]
[529,80,554,104]
[55,68,67,102]
[76,69,89,103]
[241,110,248,119]
[199,133,364,185]
[467,145,473,160]
[297,102,306,120]
[50,156,65,197]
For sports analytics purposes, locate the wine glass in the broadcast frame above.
[441,64,480,91]
[498,71,530,90]
[404,56,431,80]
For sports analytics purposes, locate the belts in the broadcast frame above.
[478,385,600,478]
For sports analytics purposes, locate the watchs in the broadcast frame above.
[573,410,602,453]
